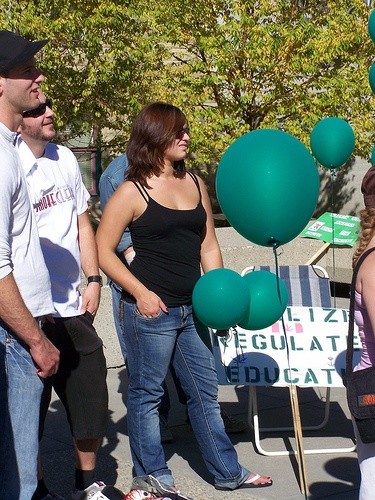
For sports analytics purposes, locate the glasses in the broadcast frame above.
[175,127,189,139]
[23,97,53,119]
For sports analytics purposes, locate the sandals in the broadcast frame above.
[215,472,272,491]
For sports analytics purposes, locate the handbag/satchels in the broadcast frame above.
[346,366,375,444]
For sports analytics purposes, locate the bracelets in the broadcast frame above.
[130,282,143,295]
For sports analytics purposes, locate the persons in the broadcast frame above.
[96,102,272,500]
[15,87,113,500]
[100,153,247,443]
[0,32,61,500]
[349,166,375,500]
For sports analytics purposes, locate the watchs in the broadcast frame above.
[87,275,103,287]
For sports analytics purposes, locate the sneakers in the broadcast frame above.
[42,491,65,500]
[71,480,121,500]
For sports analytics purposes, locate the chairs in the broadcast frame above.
[240,265,357,456]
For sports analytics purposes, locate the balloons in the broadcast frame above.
[369,62,375,95]
[214,128,320,247]
[310,118,355,169]
[236,270,288,331]
[368,9,375,45]
[191,268,250,330]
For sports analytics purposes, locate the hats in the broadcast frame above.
[0,29,49,72]
